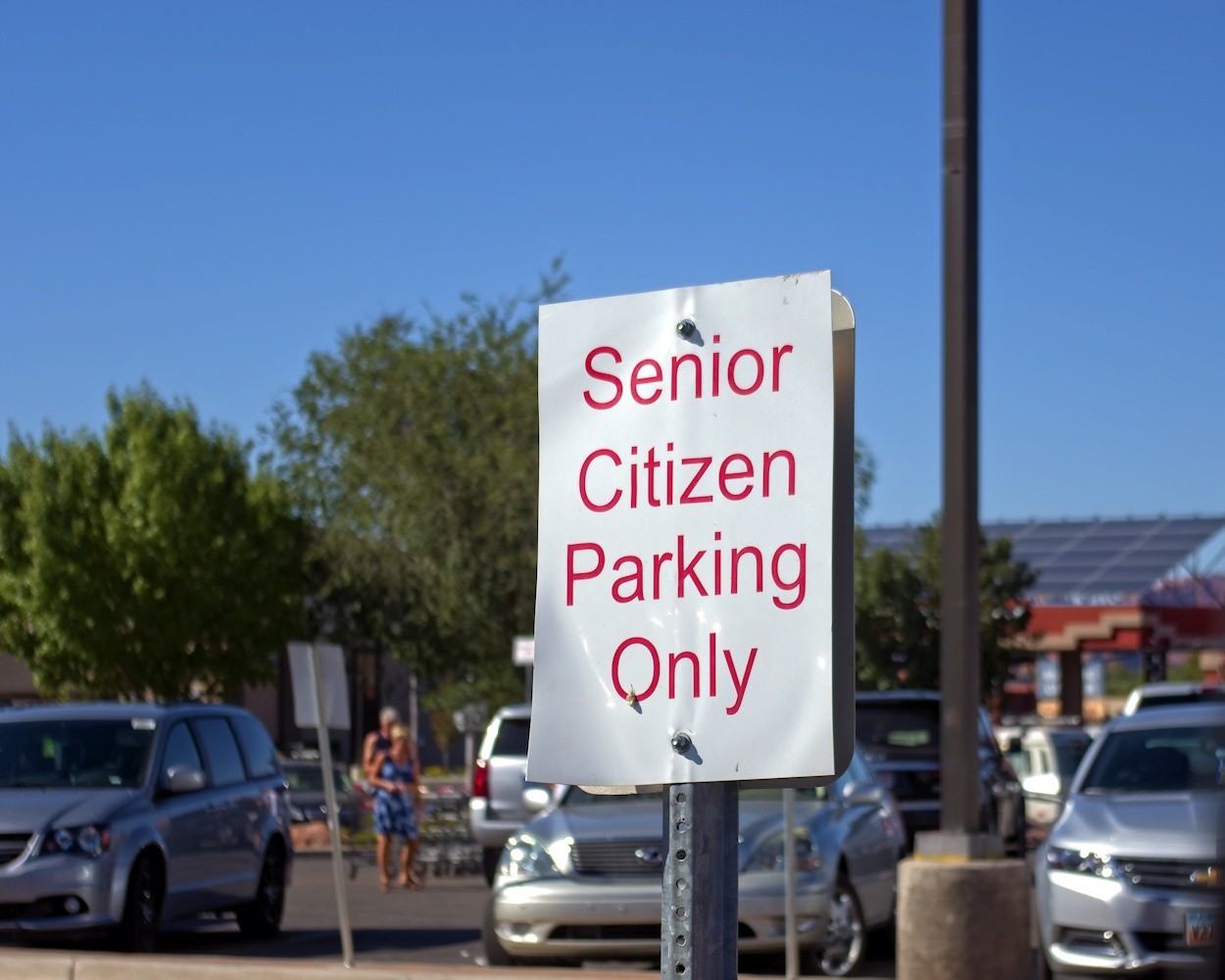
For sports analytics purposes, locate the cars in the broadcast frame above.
[279,758,365,832]
[1034,703,1225,980]
[483,726,908,977]
[1116,681,1225,717]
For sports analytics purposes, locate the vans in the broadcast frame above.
[988,719,1095,855]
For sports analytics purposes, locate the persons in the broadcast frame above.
[362,706,424,894]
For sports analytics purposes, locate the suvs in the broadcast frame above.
[468,704,567,888]
[854,687,1030,857]
[0,702,295,951]
